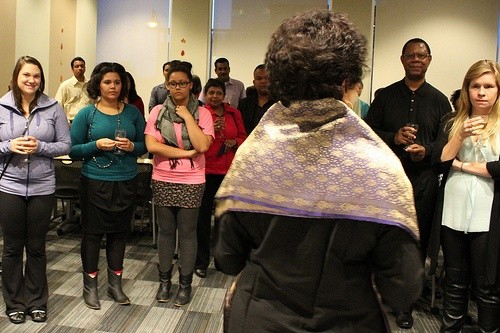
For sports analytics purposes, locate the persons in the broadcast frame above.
[431,60,500,333]
[201,58,246,106]
[214,10,423,333]
[365,38,453,329]
[194,79,248,278]
[0,56,72,324]
[149,62,206,114]
[67,62,148,310]
[121,72,145,118]
[144,63,215,307]
[238,64,280,135]
[352,80,369,120]
[55,57,98,124]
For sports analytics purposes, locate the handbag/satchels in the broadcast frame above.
[414,160,449,203]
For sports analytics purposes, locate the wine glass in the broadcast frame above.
[472,114,488,148]
[115,128,127,154]
[22,135,36,163]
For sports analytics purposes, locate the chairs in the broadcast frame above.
[131,163,153,235]
[52,159,85,235]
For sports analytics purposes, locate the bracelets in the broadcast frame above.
[461,161,465,172]
[129,142,136,154]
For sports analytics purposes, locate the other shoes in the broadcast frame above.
[29,307,47,321]
[8,310,25,323]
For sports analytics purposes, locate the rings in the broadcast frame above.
[178,105,180,108]
[229,145,231,147]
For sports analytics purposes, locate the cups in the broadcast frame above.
[217,116,225,130]
[405,123,415,143]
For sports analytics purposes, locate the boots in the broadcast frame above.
[106,267,130,304]
[175,270,193,307]
[156,263,175,303]
[81,270,101,309]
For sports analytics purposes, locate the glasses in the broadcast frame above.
[403,52,430,60]
[167,80,191,89]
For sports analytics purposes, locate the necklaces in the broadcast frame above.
[88,99,121,169]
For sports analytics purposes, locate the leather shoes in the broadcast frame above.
[195,269,207,278]
[392,309,414,328]
